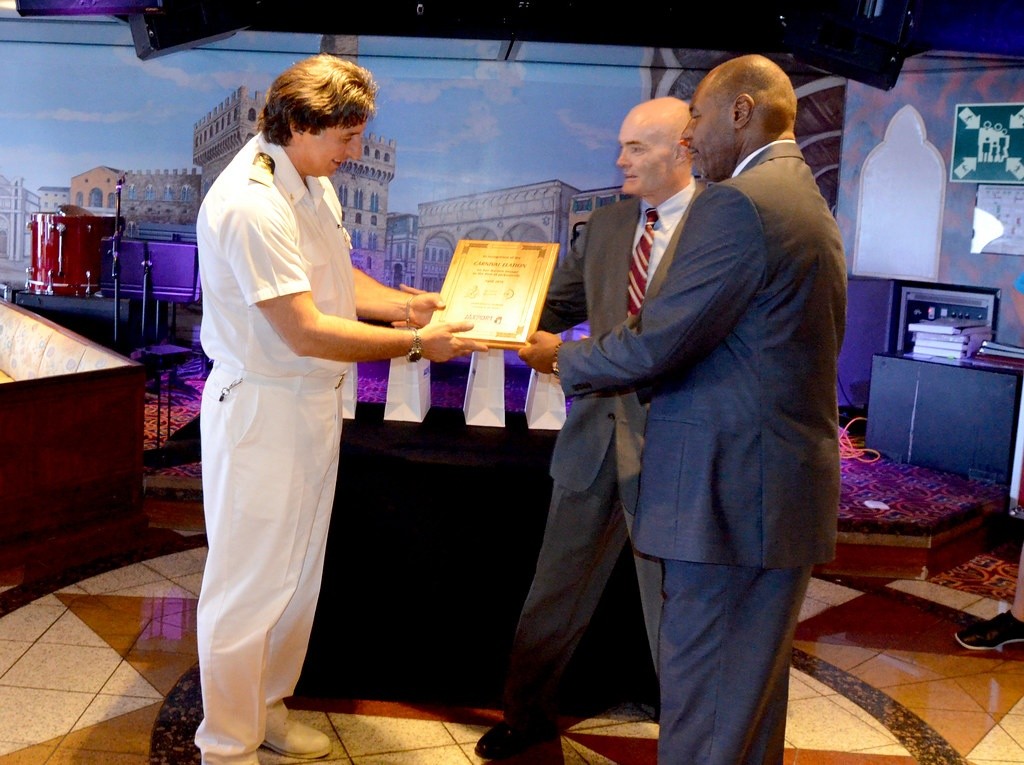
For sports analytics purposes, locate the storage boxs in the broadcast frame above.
[864,353,1024,485]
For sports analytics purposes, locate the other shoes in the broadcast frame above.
[954,613,1024,650]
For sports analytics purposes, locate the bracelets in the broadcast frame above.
[405,295,417,321]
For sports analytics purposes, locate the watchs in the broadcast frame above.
[552,343,564,378]
[406,327,422,363]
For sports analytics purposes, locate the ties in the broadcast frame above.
[626,208,658,318]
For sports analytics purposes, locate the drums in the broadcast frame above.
[26,212,104,297]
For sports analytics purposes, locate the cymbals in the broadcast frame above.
[58,204,96,216]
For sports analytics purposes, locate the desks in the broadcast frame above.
[294,402,661,705]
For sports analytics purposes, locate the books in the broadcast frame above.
[908,317,1024,367]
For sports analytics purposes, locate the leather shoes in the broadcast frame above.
[260,717,331,758]
[475,721,534,760]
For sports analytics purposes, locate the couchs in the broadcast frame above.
[0,296,145,535]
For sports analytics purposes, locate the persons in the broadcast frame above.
[517,53,846,765]
[955,456,1024,650]
[194,52,487,765]
[390,97,715,759]
